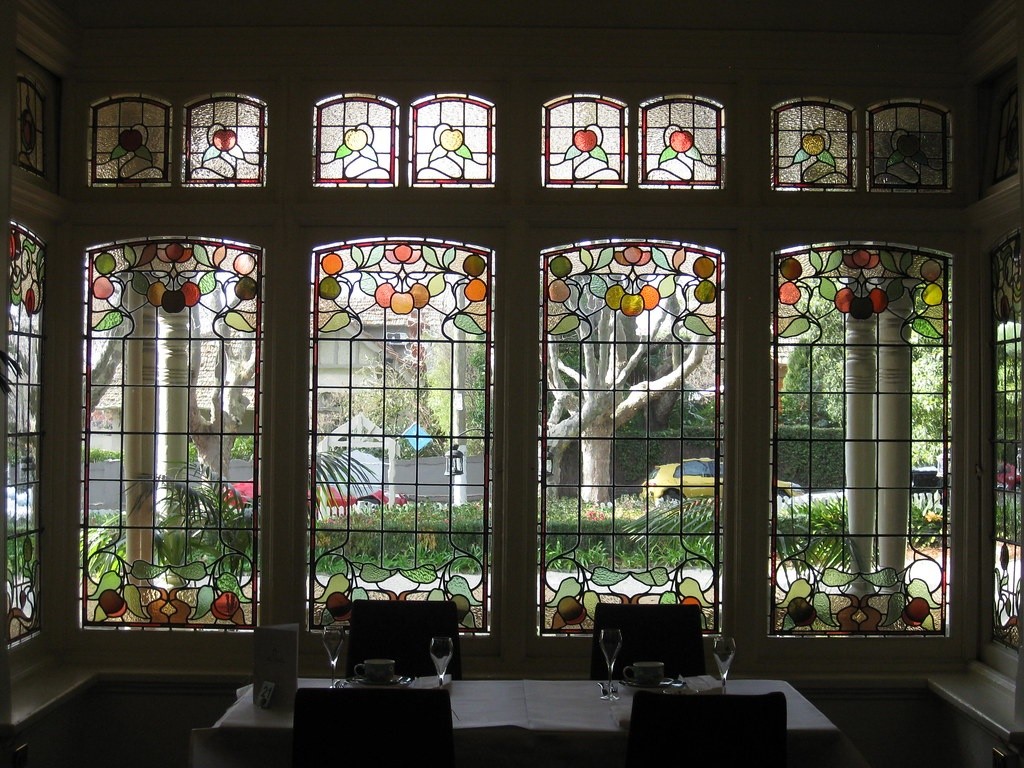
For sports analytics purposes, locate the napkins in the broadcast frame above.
[409,675,453,688]
[686,675,722,696]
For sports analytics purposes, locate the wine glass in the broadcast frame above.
[600,629,622,701]
[322,626,344,689]
[431,637,453,689]
[713,636,735,693]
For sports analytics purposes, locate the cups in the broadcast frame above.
[624,662,663,686]
[354,660,395,682]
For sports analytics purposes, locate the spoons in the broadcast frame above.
[598,682,608,696]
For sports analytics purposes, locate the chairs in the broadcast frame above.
[347,599,462,681]
[625,691,787,768]
[292,687,455,768]
[589,602,705,680]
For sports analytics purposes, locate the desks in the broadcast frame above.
[190,678,874,768]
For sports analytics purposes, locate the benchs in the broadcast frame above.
[930,671,1024,767]
[0,657,100,768]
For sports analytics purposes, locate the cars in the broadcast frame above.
[642,457,805,501]
[223,476,407,517]
[997,462,1022,491]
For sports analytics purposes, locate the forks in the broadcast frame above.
[610,684,618,693]
